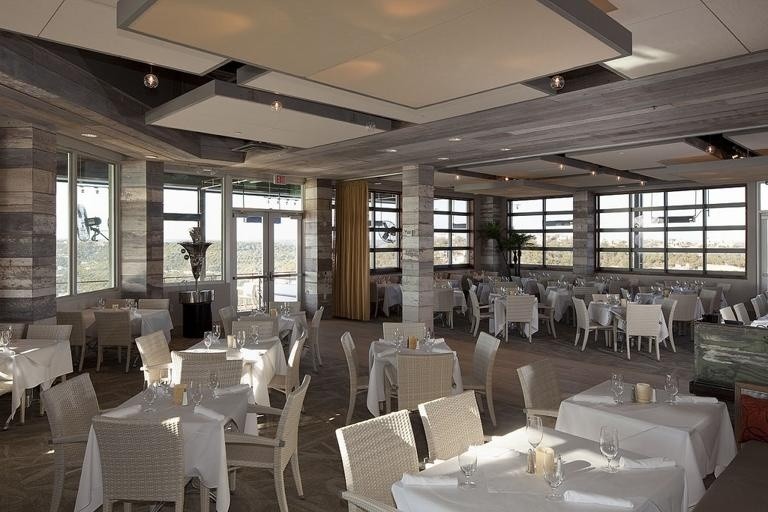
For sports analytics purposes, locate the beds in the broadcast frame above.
[515,357,576,426]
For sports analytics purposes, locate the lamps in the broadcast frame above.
[270,92,282,112]
[550,75,565,89]
[144,64,159,89]
[705,144,716,155]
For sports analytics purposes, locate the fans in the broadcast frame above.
[74,206,102,243]
[377,220,399,243]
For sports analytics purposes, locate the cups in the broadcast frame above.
[375,271,623,295]
[96,297,144,314]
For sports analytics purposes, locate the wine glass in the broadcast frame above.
[203,331,212,352]
[611,374,625,407]
[664,372,680,405]
[236,330,246,352]
[458,450,477,490]
[143,379,156,413]
[160,368,173,397]
[0,330,10,351]
[212,324,220,345]
[606,280,704,305]
[207,368,220,401]
[391,326,435,354]
[247,326,259,344]
[526,415,544,466]
[599,426,619,472]
[543,449,565,502]
[190,380,205,414]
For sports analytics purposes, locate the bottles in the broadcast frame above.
[631,383,635,401]
[651,385,657,403]
[232,337,236,349]
[182,387,188,406]
[527,449,534,473]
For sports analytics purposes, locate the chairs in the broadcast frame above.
[38,370,100,510]
[370,270,768,360]
[0,295,326,418]
[88,413,198,510]
[459,329,502,426]
[396,351,454,408]
[331,407,419,510]
[417,387,496,469]
[220,373,312,512]
[340,329,391,424]
